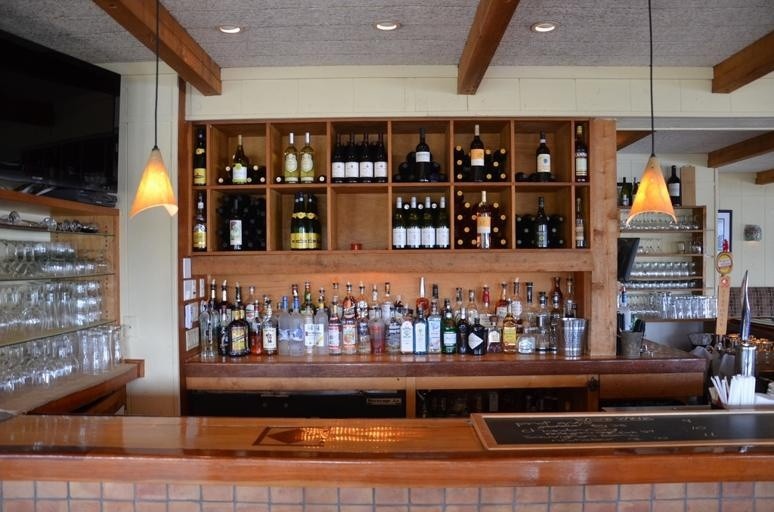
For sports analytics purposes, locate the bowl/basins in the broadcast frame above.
[687,332,714,345]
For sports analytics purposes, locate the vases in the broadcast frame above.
[744,224,762,240]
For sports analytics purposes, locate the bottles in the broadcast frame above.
[620,176,629,206]
[632,177,640,206]
[197,275,577,363]
[665,165,681,207]
[417,391,573,418]
[191,125,590,253]
[258,400,290,417]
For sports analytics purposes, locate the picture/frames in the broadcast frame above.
[717,209,733,254]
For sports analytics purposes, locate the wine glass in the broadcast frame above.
[0,211,123,396]
[620,210,717,320]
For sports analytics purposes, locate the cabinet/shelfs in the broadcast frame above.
[0,189,145,414]
[178,116,717,417]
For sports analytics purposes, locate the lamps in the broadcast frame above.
[128,0,179,219]
[627,0,677,229]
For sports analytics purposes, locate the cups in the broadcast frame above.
[558,317,587,360]
[619,331,642,359]
[721,333,774,372]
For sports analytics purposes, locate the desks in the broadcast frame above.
[727,316,774,338]
